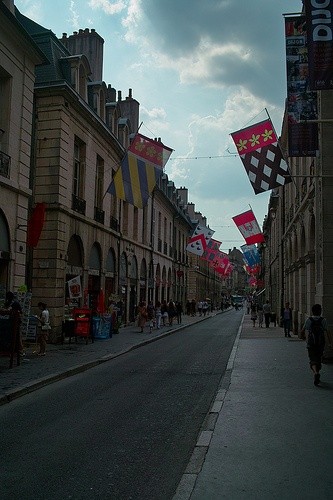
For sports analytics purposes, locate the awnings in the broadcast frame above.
[255,288,266,296]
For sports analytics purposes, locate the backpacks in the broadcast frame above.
[284,307,290,320]
[156,306,162,316]
[306,316,325,352]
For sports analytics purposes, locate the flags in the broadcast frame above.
[106,134,173,209]
[68,276,82,299]
[231,119,293,194]
[240,244,261,287]
[200,239,234,277]
[232,210,265,245]
[185,223,215,256]
[26,203,46,247]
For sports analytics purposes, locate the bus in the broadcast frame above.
[231,294,242,307]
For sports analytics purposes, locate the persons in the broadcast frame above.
[282,302,293,337]
[137,299,230,333]
[235,299,271,328]
[34,302,50,355]
[2,292,26,357]
[301,304,332,385]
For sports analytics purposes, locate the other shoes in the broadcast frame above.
[37,352,46,356]
[157,326,160,329]
[150,326,153,333]
[314,373,321,386]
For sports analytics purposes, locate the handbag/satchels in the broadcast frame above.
[41,322,52,331]
